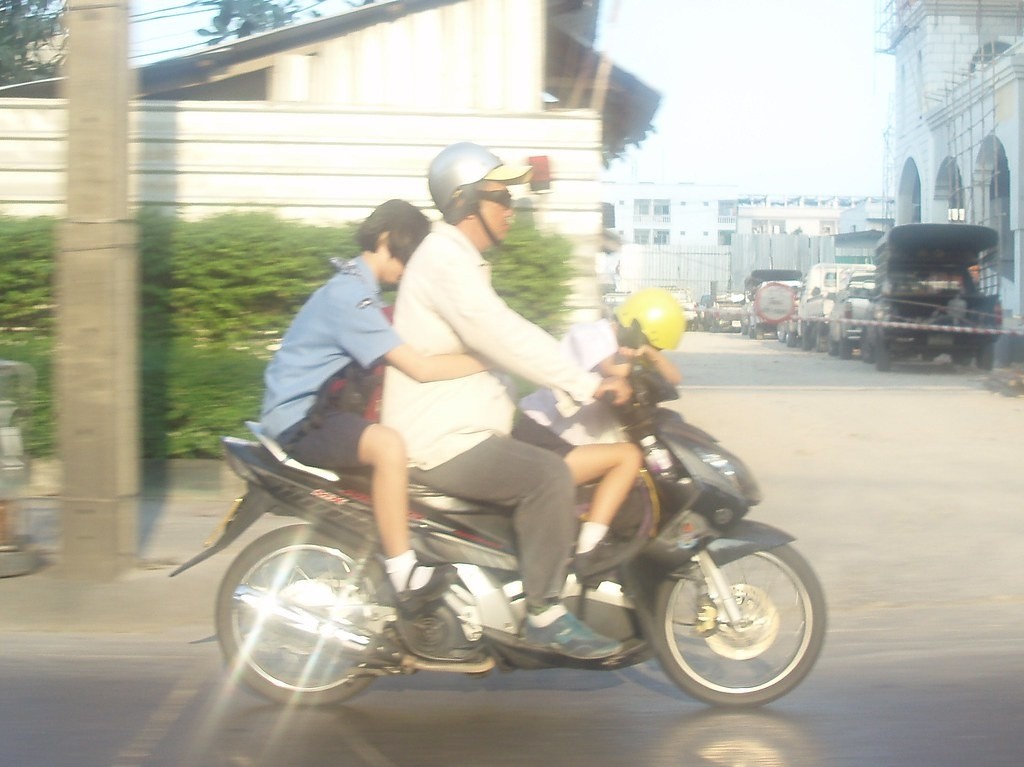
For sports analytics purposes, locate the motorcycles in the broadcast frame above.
[166,318,831,715]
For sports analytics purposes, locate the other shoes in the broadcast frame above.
[573,534,648,583]
[515,612,623,660]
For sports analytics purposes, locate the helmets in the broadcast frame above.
[428,142,503,212]
[614,288,687,348]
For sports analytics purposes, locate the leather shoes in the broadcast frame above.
[396,561,458,613]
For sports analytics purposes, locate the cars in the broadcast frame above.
[668,263,879,360]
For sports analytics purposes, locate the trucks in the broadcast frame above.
[867,222,1007,370]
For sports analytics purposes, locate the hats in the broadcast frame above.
[451,164,536,198]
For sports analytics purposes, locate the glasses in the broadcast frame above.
[477,189,511,206]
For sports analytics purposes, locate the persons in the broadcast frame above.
[512,287,685,583]
[259,198,459,618]
[379,144,632,660]
